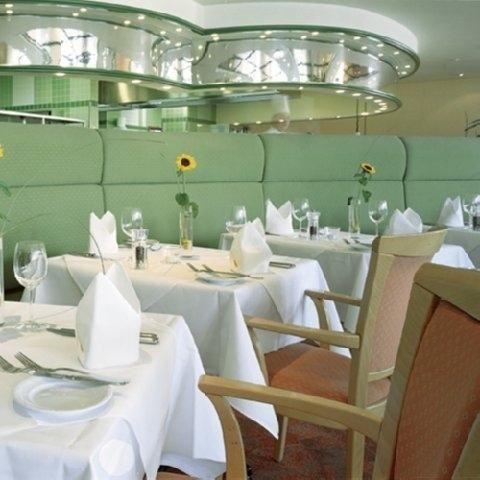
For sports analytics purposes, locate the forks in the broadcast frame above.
[186,262,251,278]
[0,352,131,387]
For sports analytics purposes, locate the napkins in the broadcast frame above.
[73,259,141,370]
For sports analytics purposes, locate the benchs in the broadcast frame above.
[1,121,479,293]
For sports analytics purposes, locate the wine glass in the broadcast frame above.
[120,205,143,265]
[292,196,310,241]
[367,199,388,241]
[12,238,51,333]
[461,191,480,233]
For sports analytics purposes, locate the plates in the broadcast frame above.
[194,269,244,287]
[11,369,115,422]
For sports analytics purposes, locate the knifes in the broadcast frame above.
[45,327,159,345]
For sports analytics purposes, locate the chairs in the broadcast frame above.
[242,223,450,423]
[139,262,478,478]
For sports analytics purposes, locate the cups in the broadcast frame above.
[323,226,341,242]
[131,227,152,271]
[224,205,247,239]
[306,212,321,242]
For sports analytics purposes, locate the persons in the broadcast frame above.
[263,95,291,135]
